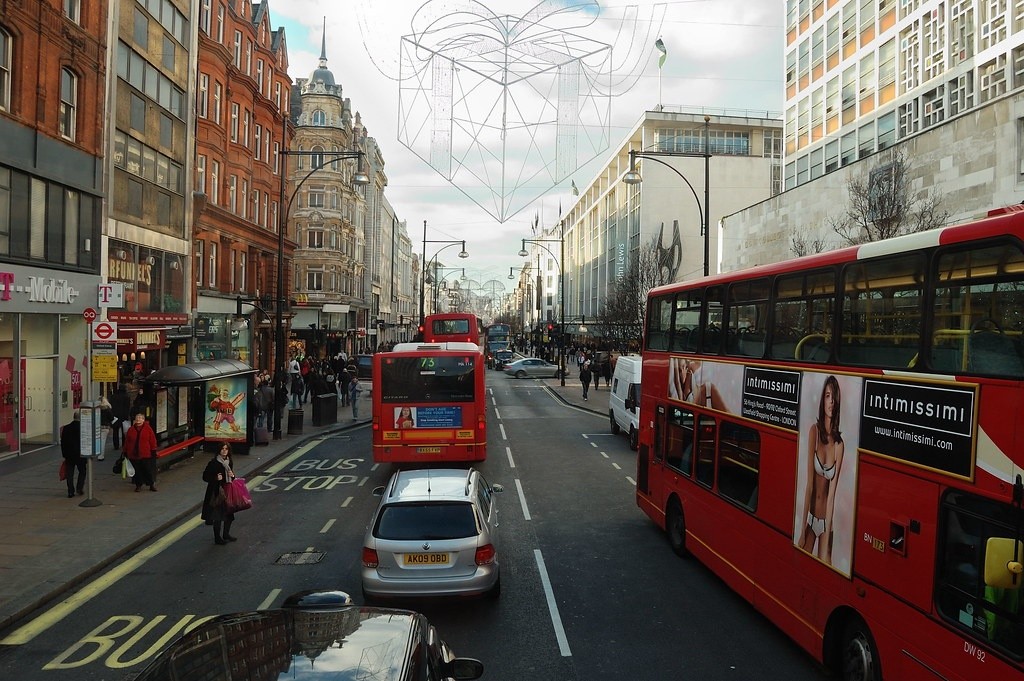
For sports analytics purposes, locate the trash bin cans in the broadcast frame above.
[596,352,602,362]
[288,409,304,435]
[312,393,338,427]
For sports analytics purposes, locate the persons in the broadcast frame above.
[123,411,158,492]
[61,412,87,498]
[519,338,617,391]
[201,441,237,544]
[359,334,417,354]
[798,376,845,566]
[97,387,149,461]
[396,407,414,428]
[254,349,364,433]
[673,358,727,413]
[579,364,591,401]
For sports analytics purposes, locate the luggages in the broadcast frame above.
[254,416,269,446]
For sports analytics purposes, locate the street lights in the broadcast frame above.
[230,111,370,439]
[518,219,587,386]
[513,280,534,357]
[397,220,469,342]
[623,116,715,324]
[508,254,542,358]
[434,254,466,313]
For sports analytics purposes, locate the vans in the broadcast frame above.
[610,352,642,448]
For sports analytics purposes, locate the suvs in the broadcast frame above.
[361,467,505,603]
[494,349,513,369]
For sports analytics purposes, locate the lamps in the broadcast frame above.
[146,248,155,265]
[116,242,127,259]
[170,254,178,270]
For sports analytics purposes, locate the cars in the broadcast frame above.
[345,354,373,381]
[130,589,484,681]
[505,357,569,378]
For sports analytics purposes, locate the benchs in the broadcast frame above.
[156,435,205,459]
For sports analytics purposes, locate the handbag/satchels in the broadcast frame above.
[294,361,299,370]
[215,478,252,513]
[59,461,66,481]
[113,452,135,480]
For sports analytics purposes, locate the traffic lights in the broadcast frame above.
[418,325,424,342]
[548,325,552,337]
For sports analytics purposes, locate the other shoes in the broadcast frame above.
[584,399,587,400]
[150,482,158,490]
[122,420,131,437]
[135,486,141,491]
[352,417,357,421]
[77,489,83,495]
[69,493,74,497]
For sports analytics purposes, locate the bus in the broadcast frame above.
[636,203,1024,681]
[486,322,512,354]
[424,314,488,355]
[372,343,486,462]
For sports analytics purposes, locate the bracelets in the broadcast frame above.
[232,474,234,477]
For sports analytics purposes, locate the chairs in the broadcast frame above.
[647,319,1024,377]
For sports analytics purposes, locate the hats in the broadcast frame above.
[343,369,347,372]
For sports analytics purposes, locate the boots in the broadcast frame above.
[213,520,237,545]
